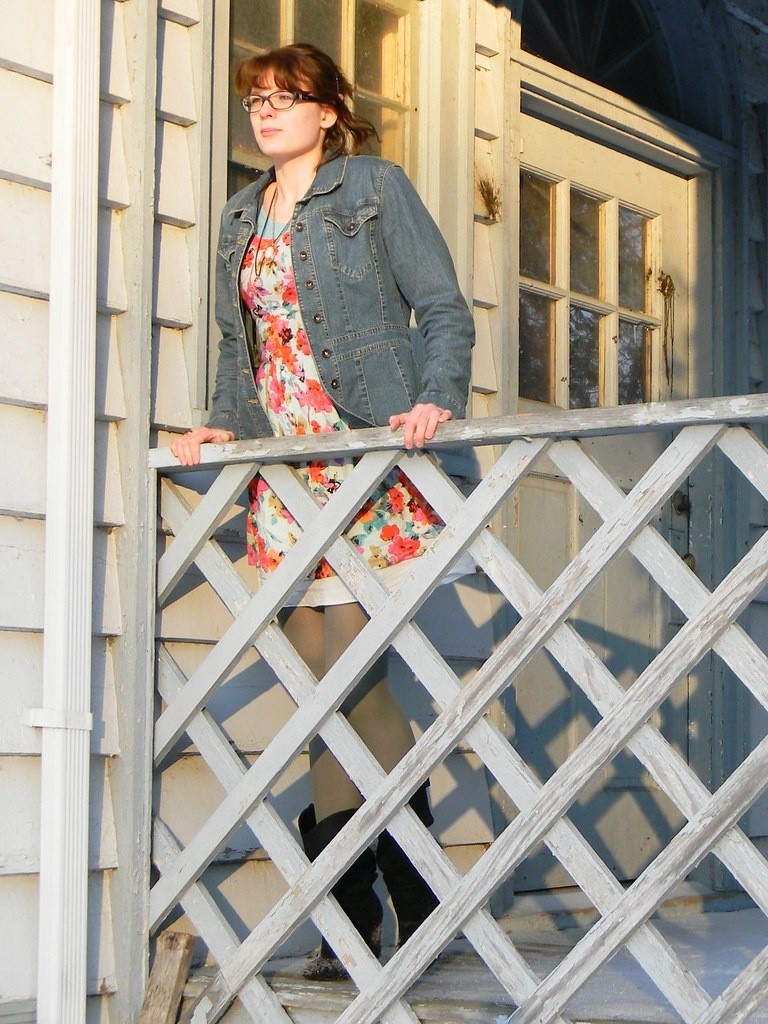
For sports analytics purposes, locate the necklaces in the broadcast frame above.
[252,188,292,277]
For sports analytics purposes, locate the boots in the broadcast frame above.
[378,776,440,968]
[298,803,383,980]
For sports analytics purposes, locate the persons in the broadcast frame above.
[170,42,476,979]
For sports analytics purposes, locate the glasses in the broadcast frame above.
[241,91,322,113]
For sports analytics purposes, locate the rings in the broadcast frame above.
[186,430,192,435]
